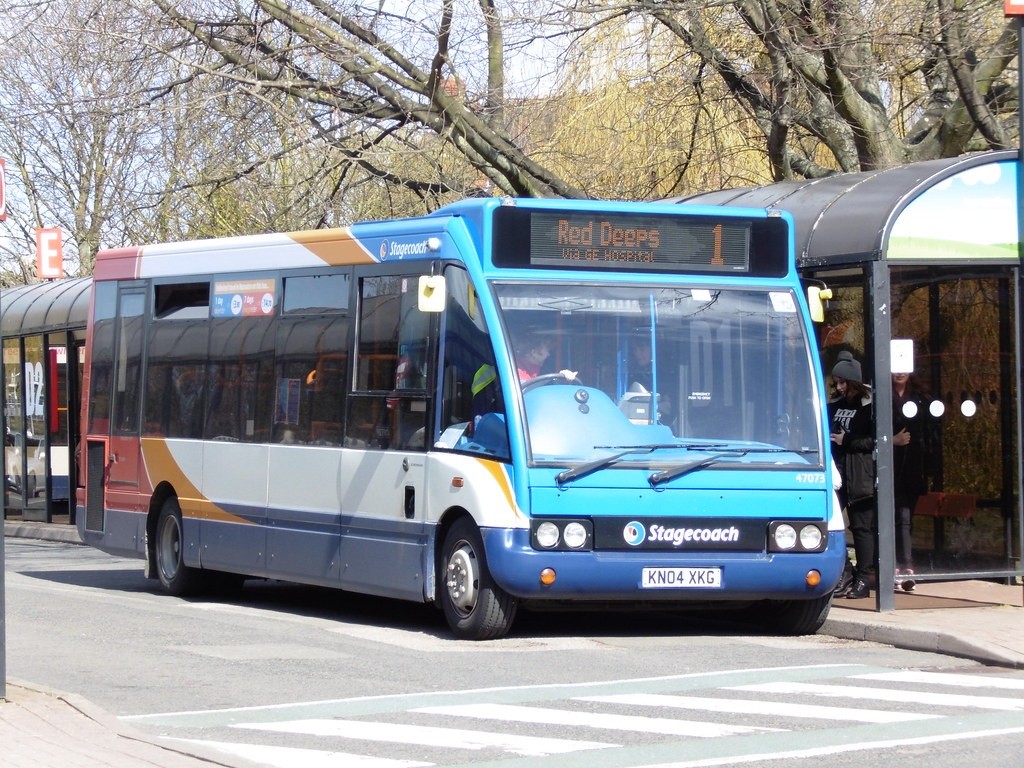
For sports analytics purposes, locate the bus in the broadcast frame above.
[74,195,845,640]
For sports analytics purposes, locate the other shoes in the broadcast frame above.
[901,578,914,592]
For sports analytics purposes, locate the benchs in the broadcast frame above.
[916,490,977,560]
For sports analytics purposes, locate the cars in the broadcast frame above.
[0,432,45,498]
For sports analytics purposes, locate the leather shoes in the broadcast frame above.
[847,579,869,598]
[834,569,854,596]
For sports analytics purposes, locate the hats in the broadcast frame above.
[832,351,862,382]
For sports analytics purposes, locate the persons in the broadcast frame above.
[472,329,678,421]
[827,352,942,600]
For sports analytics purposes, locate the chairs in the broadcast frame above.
[470,364,499,420]
